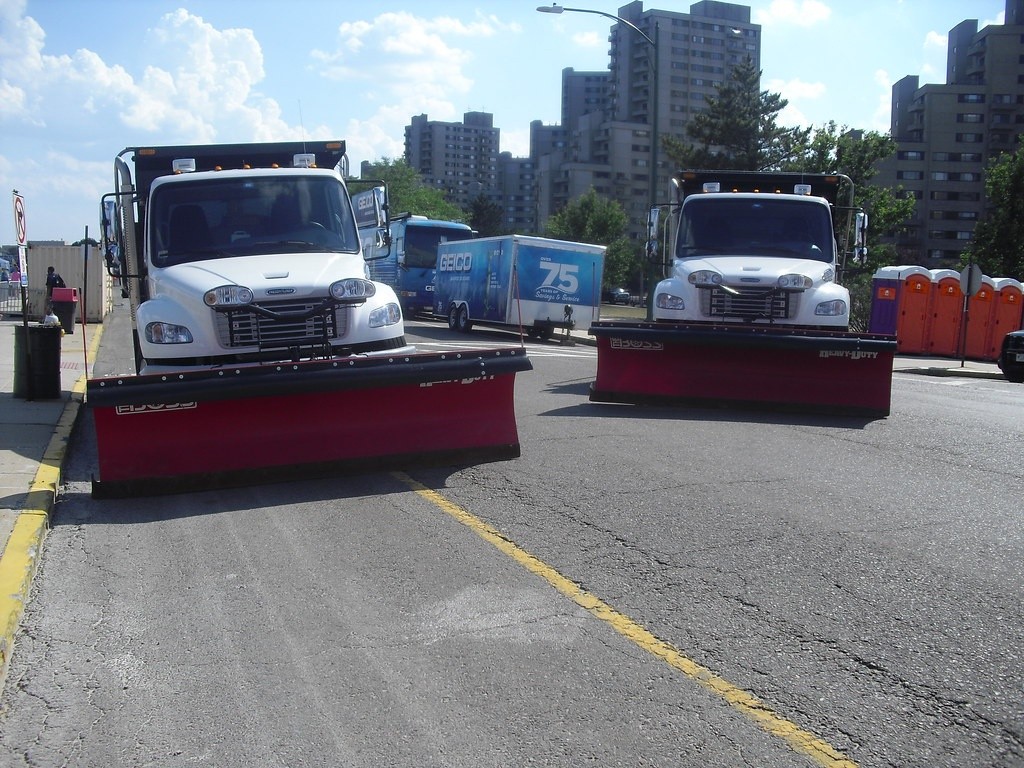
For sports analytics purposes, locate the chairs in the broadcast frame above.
[164,204,215,254]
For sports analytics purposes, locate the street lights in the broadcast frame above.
[536,4,663,322]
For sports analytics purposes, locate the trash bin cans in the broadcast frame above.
[50,288,78,334]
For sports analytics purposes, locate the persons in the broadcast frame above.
[14,264,18,271]
[44,303,59,324]
[1,268,8,281]
[46,266,66,297]
[11,267,20,288]
[769,216,828,262]
[10,266,14,272]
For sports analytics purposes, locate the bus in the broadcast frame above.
[354,211,481,320]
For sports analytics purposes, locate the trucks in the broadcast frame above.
[85,139,539,501]
[585,169,901,422]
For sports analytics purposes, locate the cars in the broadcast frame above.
[608,287,631,305]
